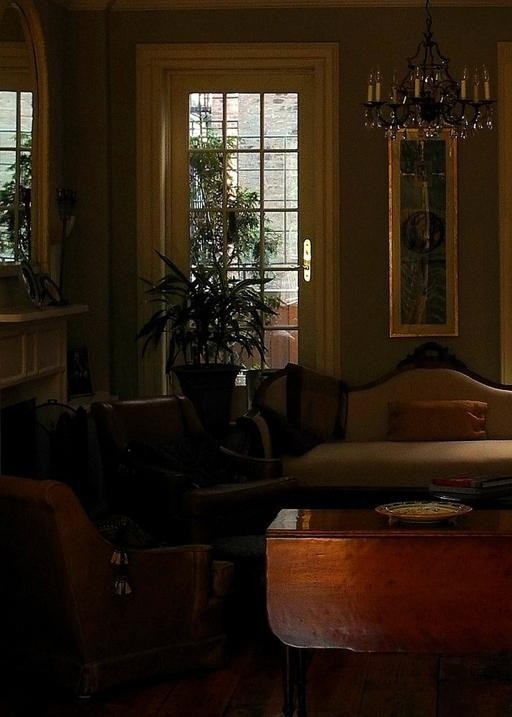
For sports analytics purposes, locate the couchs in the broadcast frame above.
[252,342,512,508]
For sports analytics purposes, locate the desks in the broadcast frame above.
[264,507,512,716]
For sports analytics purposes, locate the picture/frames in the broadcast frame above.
[388,129,459,337]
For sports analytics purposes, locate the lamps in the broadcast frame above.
[359,2,496,142]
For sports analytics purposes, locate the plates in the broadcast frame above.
[403,210,445,255]
[374,499,474,527]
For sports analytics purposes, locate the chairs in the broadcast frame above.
[92,395,297,544]
[1,473,228,708]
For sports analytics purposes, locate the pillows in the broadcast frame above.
[256,405,323,456]
[389,399,489,442]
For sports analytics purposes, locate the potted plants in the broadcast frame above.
[136,248,278,438]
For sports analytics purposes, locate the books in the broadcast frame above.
[428,471,512,499]
